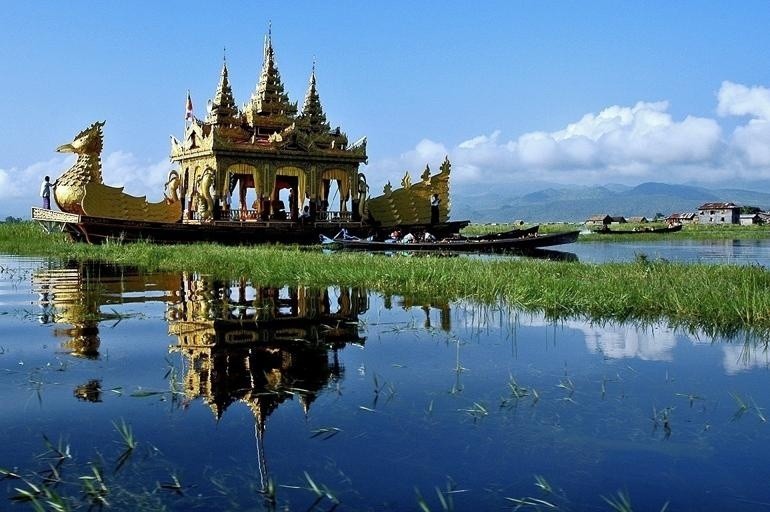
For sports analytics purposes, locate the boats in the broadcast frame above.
[338,248,579,264]
[593,220,682,234]
[458,223,542,238]
[322,228,587,254]
[30,22,477,241]
[30,264,450,440]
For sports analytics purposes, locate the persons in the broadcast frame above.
[297,204,313,222]
[429,193,441,223]
[631,219,683,233]
[39,174,59,210]
[377,212,541,249]
